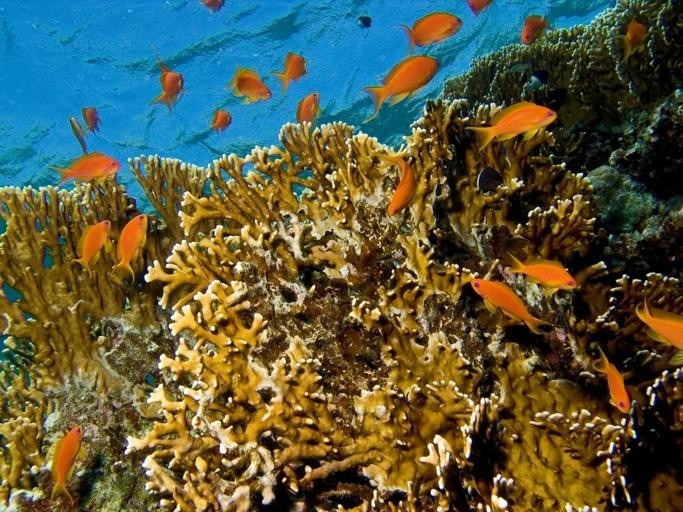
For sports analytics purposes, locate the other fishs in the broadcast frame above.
[49,152,150,285]
[43,424,84,507]
[146,51,322,131]
[614,19,649,64]
[593,295,683,415]
[374,152,426,218]
[360,11,465,126]
[464,100,558,153]
[470,249,575,335]
[521,10,556,46]
[82,105,102,137]
